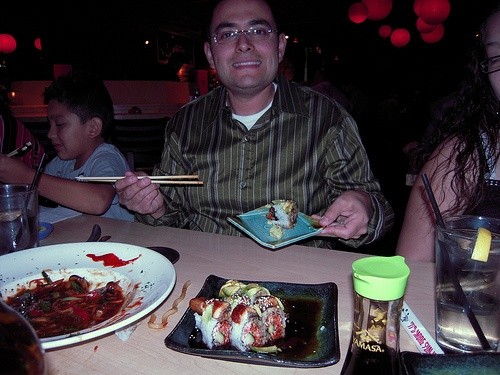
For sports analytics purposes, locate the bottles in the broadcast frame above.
[0,299,47,375]
[340,256,411,375]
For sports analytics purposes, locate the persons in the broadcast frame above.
[0,71,135,222]
[115,0,395,252]
[395,0,500,263]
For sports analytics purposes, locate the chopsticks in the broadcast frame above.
[74,175,203,184]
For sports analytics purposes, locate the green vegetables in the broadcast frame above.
[16,270,119,335]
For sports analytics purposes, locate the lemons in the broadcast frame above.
[471,227,492,263]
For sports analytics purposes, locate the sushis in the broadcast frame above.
[189,278,287,352]
[267,199,299,229]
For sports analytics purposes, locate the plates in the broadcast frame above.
[0,242,177,352]
[164,274,341,368]
[37,221,53,239]
[227,205,325,249]
[147,246,179,264]
[401,350,500,375]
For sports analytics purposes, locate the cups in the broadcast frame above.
[434,216,500,353]
[0,183,41,255]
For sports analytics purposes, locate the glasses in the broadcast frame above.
[477,55,500,74]
[207,26,280,45]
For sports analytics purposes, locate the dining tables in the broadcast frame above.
[36,214,435,375]
